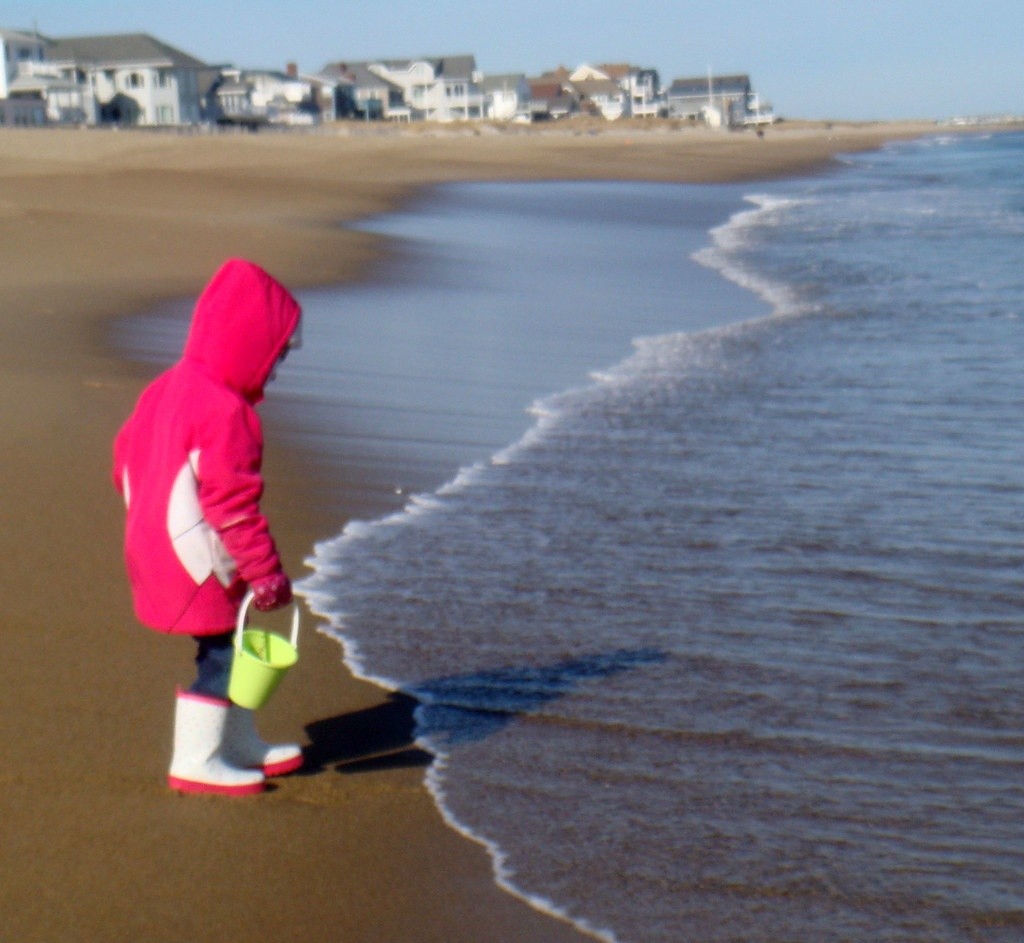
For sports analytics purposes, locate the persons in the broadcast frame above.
[112,258,305,797]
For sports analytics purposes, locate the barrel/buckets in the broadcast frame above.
[226,588,299,710]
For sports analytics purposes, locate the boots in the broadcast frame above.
[165,683,266,797]
[223,697,304,778]
